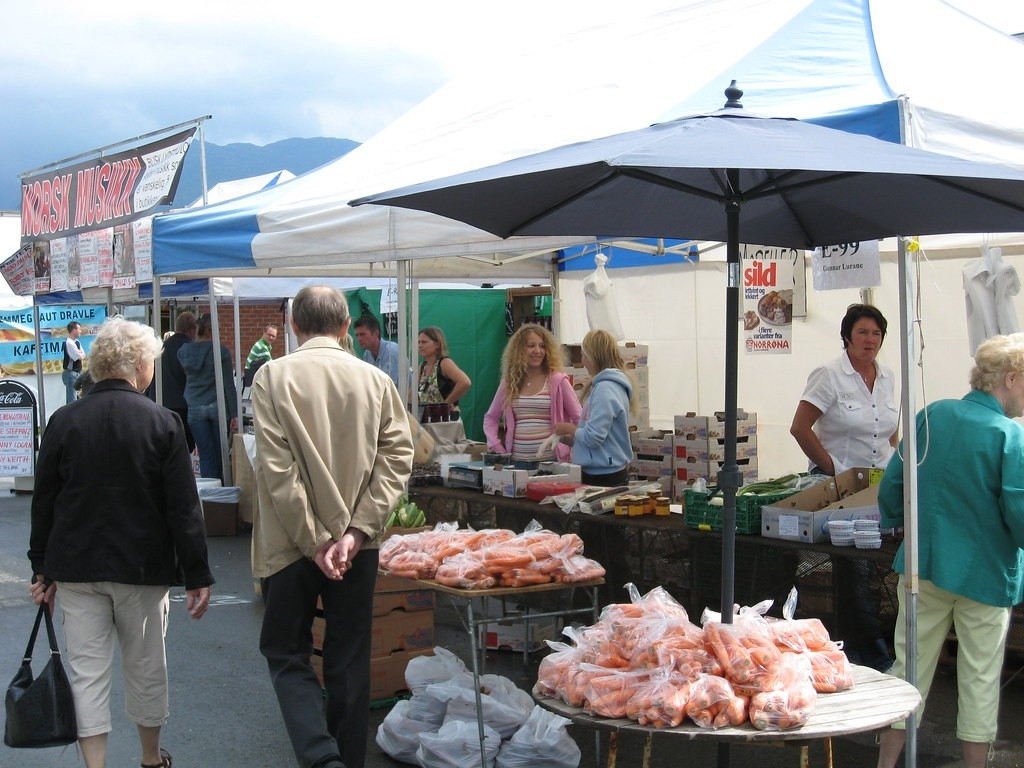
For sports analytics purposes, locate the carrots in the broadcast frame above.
[536,604,852,729]
[378,528,605,587]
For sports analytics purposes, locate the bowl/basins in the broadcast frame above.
[482,453,511,465]
[829,520,881,549]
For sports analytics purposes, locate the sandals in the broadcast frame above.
[142,747,171,768]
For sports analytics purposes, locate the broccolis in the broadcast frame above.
[397,503,426,527]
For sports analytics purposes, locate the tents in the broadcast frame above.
[150,0,1024,768]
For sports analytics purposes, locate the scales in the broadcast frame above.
[447,460,516,491]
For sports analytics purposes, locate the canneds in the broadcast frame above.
[614,490,671,519]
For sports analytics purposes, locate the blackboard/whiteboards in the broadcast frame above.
[0,406,35,478]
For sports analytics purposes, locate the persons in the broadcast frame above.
[251,284,415,768]
[555,330,635,609]
[28,317,216,768]
[790,304,901,477]
[878,331,1024,768]
[417,327,472,423]
[482,322,583,471]
[61,322,84,405]
[176,312,239,479]
[245,325,277,369]
[161,311,196,456]
[352,315,398,385]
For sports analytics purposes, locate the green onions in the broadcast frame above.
[738,474,797,494]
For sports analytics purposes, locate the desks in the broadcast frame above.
[408,482,908,663]
[532,661,923,768]
[377,566,611,768]
[232,433,256,524]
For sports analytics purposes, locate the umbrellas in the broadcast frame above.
[352,78,1024,625]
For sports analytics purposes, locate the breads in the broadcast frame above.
[0,359,64,376]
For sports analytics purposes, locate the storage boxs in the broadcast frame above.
[416,333,905,549]
[478,613,564,653]
[311,522,432,702]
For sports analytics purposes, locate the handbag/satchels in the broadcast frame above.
[536,579,853,728]
[375,647,582,768]
[378,518,607,588]
[3,573,78,748]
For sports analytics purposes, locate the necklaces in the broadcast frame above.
[527,382,531,386]
